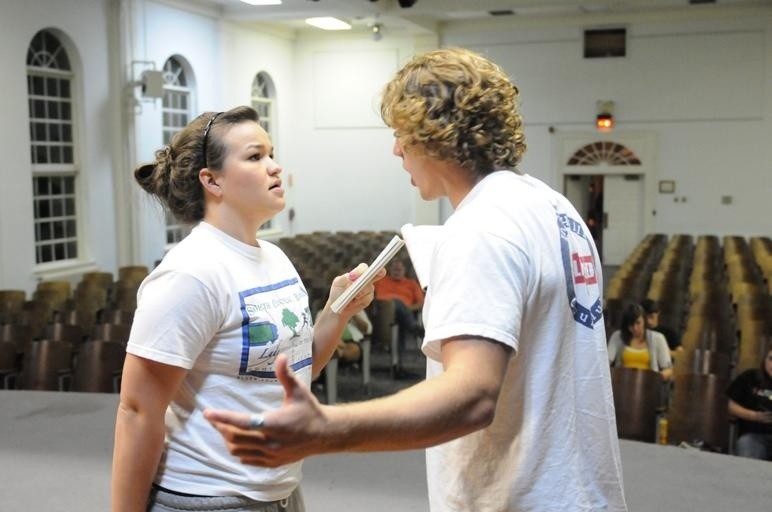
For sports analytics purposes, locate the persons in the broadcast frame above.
[312,256,426,394]
[202,45,627,512]
[606,303,674,426]
[725,349,772,461]
[110,106,386,511]
[639,297,685,364]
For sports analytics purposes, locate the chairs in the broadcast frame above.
[1,228,423,404]
[608,235,768,456]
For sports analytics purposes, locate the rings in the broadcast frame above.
[251,412,265,431]
